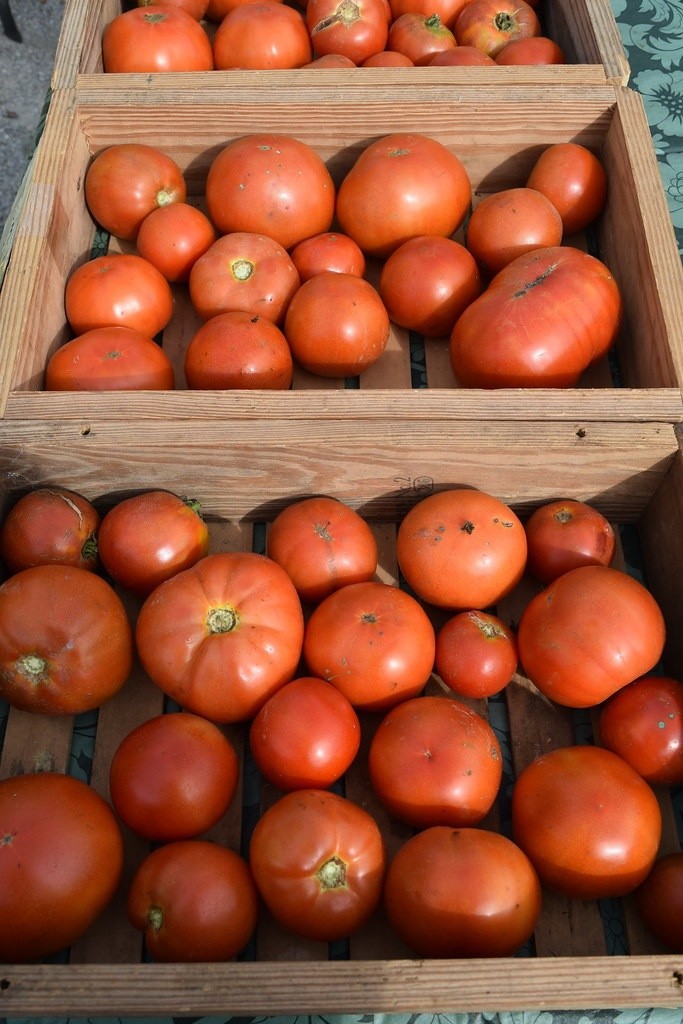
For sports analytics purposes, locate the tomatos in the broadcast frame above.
[45,133,623,390]
[0,488,682,961]
[100,0,567,75]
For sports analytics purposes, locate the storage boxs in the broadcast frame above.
[2,0,683,1008]
